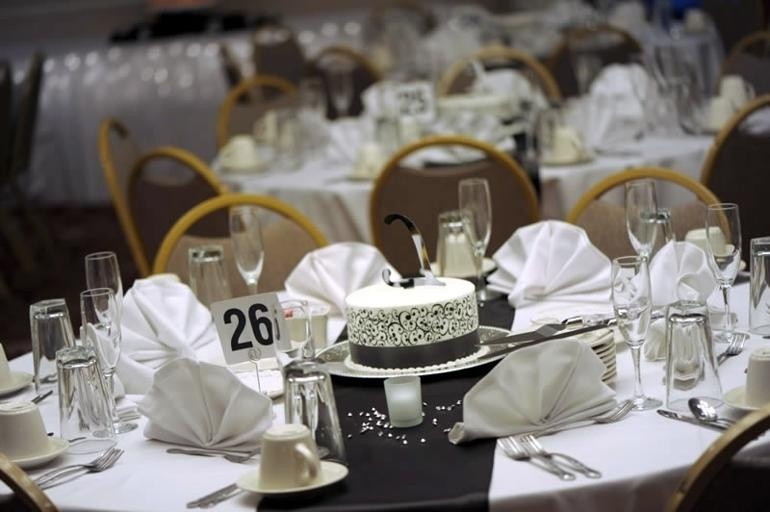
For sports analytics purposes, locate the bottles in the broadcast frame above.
[86,253,124,348]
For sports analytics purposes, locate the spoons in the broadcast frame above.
[689,398,766,432]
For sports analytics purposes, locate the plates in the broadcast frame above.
[238,460,349,493]
[0,370,31,396]
[724,383,768,414]
[9,437,70,473]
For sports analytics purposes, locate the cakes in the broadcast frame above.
[345,273,479,371]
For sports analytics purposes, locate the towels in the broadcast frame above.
[138,356,273,457]
[487,220,617,307]
[628,236,715,309]
[117,271,213,364]
[448,339,617,448]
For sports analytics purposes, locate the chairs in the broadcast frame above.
[700,92,770,274]
[92,20,379,300]
[566,166,731,276]
[667,403,769,512]
[439,17,770,121]
[369,137,539,279]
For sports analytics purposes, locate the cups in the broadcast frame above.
[640,209,675,258]
[30,296,73,398]
[12,1,757,208]
[189,246,229,304]
[55,345,122,438]
[751,239,770,338]
[663,301,723,409]
[257,424,325,486]
[748,348,769,408]
[436,211,481,278]
[0,345,11,381]
[282,360,347,461]
[0,406,51,453]
[270,300,314,376]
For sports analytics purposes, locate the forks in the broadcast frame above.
[499,434,600,482]
[167,448,259,465]
[33,446,124,490]
[657,410,757,445]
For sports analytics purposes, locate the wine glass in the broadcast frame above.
[622,177,660,263]
[78,287,137,438]
[611,257,660,410]
[705,201,751,344]
[229,205,266,296]
[456,176,501,304]
